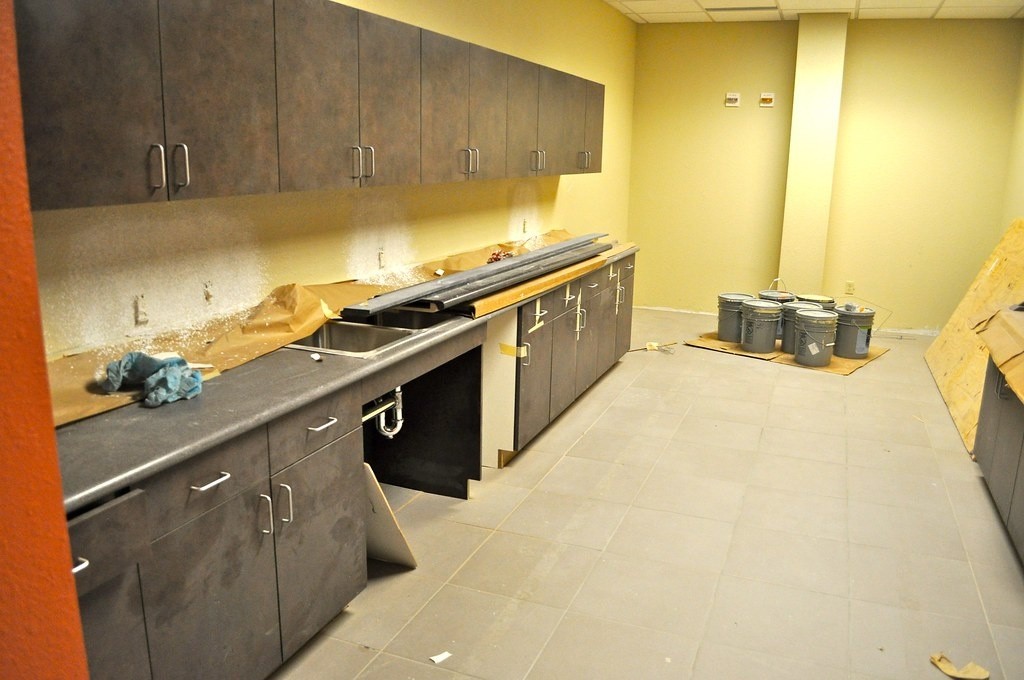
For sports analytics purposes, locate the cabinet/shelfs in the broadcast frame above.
[549,268,601,424]
[275,0,421,193]
[138,378,368,680]
[565,73,604,175]
[1007,440,1023,568]
[507,53,565,179]
[419,28,505,186]
[65,487,154,679]
[514,291,553,451]
[596,253,634,380]
[971,354,1023,526]
[14,0,278,212]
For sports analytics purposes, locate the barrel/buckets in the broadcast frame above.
[717,292,756,343]
[740,299,782,353]
[759,277,796,339]
[833,304,875,359]
[797,294,837,311]
[781,301,823,354]
[794,309,838,367]
